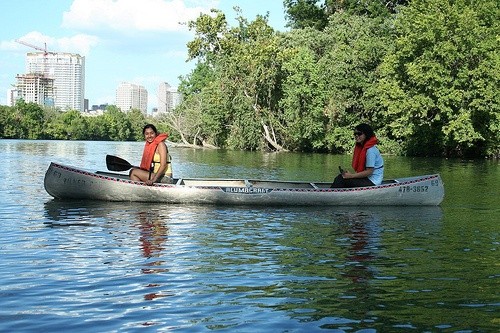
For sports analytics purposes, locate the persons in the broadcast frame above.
[130,124,172,185]
[330,123,384,188]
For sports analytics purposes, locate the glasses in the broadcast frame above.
[354,132,363,137]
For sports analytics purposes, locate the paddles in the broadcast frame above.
[106,154,147,172]
[337,165,345,174]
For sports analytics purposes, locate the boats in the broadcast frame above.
[44,162,446,206]
